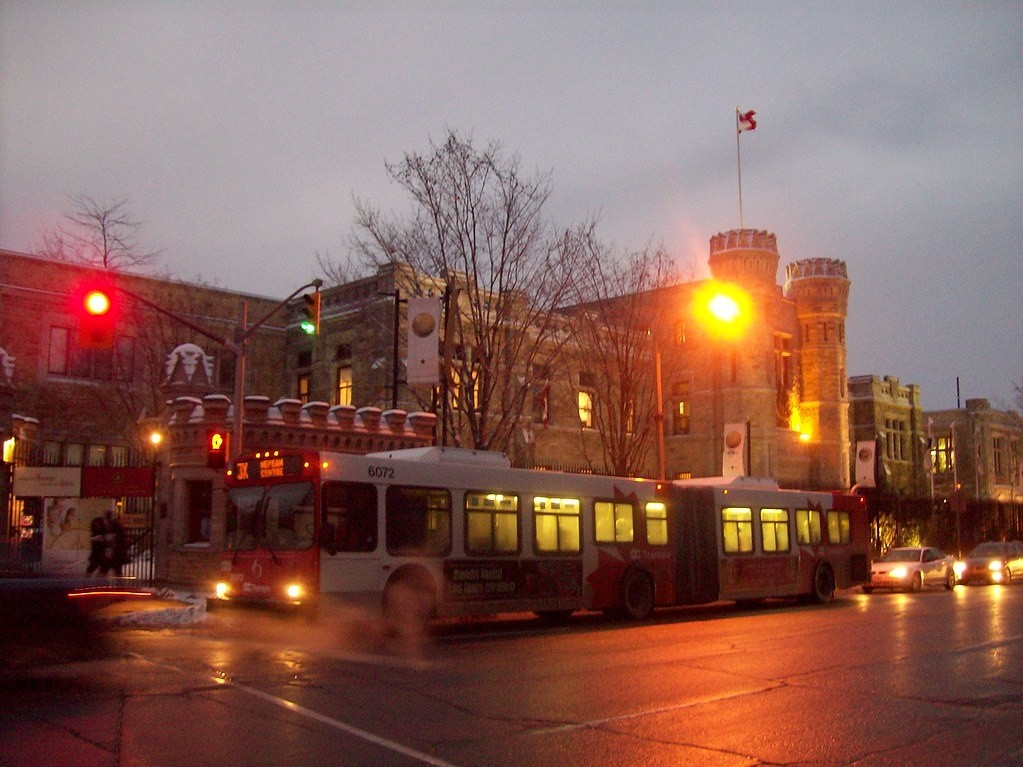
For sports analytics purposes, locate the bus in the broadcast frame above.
[218,447,871,642]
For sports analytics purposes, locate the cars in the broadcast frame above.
[864,545,957,591]
[957,541,1023,584]
[0,537,41,568]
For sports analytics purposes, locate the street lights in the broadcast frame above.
[654,291,746,479]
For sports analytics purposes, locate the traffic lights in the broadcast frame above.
[206,432,229,467]
[81,286,115,348]
[298,292,321,335]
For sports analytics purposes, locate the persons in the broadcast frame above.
[46,498,80,549]
[85,510,132,577]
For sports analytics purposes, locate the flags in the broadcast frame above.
[737,110,757,133]
